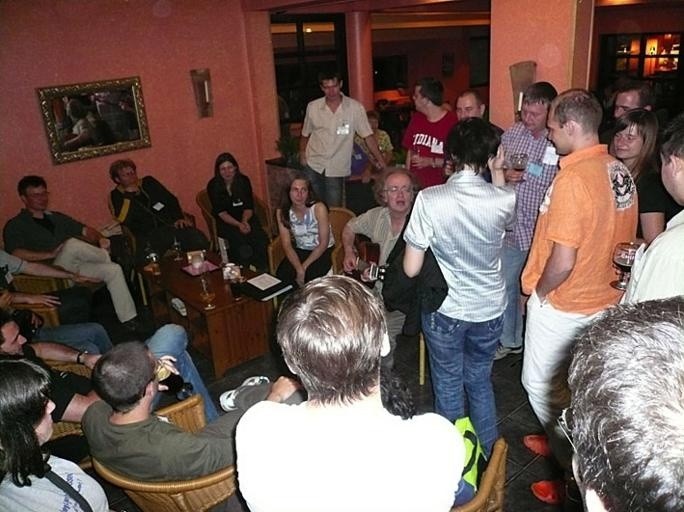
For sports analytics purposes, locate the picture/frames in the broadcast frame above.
[33,72,154,167]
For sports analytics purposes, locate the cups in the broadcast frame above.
[227,271,242,301]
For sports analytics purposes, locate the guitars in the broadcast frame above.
[341,234,391,288]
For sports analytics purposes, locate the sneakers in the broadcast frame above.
[533,481,566,505]
[525,434,551,456]
[494,344,523,360]
[219,376,270,413]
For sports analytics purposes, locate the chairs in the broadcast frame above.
[191,182,273,266]
[92,389,239,511]
[0,247,91,511]
[259,206,356,286]
[451,433,517,512]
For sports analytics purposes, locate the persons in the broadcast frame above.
[1,250,220,511]
[207,152,269,274]
[2,144,156,333]
[235,70,683,512]
[109,159,208,273]
[81,340,305,482]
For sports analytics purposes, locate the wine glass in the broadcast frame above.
[608,241,640,292]
[510,152,529,184]
[171,243,184,261]
[159,372,193,400]
[198,275,216,311]
[146,253,162,277]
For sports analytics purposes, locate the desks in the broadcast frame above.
[137,249,282,379]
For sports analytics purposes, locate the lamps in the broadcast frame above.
[190,66,213,117]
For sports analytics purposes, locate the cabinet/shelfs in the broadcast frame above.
[262,156,379,217]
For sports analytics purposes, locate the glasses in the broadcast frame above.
[384,187,412,193]
